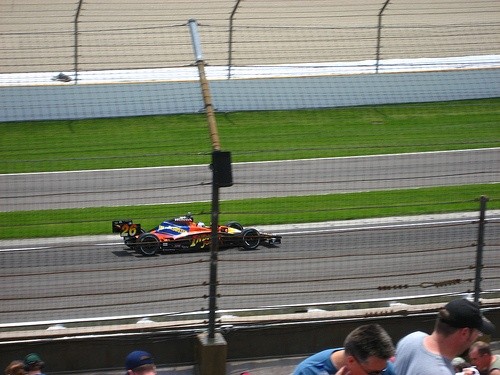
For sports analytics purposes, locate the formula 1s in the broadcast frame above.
[112,215,282,257]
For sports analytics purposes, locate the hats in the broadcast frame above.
[125,351,153,370]
[439,299,496,334]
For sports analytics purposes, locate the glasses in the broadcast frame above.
[355,357,389,375]
